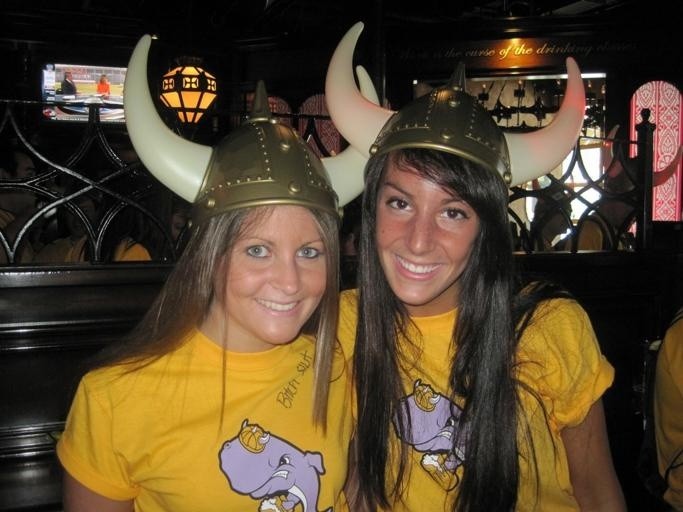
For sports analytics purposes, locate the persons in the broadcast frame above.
[0,147,155,262]
[575,156,639,251]
[52,203,364,512]
[527,188,572,252]
[331,148,629,511]
[652,305,683,511]
[60,72,77,94]
[339,206,362,291]
[96,74,110,94]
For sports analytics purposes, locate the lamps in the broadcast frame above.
[157,52,217,140]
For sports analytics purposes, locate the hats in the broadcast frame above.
[325,19,586,192]
[123,32,383,227]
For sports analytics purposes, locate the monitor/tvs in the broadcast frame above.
[38,59,129,127]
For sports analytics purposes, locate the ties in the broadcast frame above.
[71,80,74,88]
